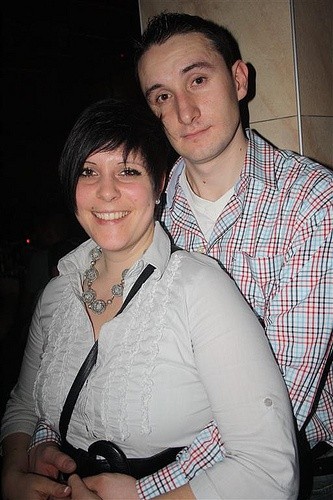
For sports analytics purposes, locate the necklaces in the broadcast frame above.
[80,247,131,313]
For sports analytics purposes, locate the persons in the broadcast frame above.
[2,100,298,500]
[26,12,333,499]
